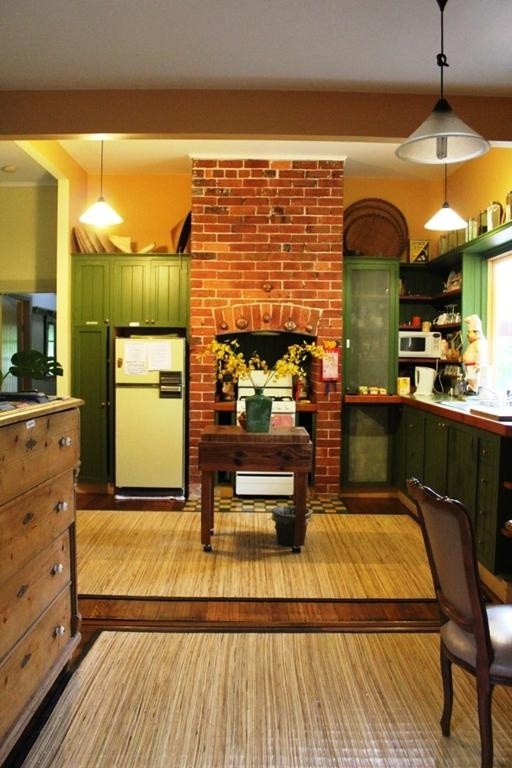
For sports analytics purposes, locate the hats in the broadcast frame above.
[464,314,482,331]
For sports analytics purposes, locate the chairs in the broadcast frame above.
[405,476,512,767]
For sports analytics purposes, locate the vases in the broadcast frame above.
[245,387,272,432]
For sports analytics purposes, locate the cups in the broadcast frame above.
[422,321,431,332]
[412,316,421,326]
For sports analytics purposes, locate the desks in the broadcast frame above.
[197,425,315,554]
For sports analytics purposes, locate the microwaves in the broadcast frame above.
[397,332,441,358]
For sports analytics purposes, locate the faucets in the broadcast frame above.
[505,390,512,408]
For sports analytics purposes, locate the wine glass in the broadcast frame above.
[444,305,451,323]
[449,304,458,323]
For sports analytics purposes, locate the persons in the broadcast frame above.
[459,315,488,397]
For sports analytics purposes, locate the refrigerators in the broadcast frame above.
[114,337,185,502]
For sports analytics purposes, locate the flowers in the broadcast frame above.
[197,340,337,389]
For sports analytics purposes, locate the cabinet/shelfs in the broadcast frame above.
[400,248,464,364]
[399,397,424,518]
[477,417,512,603]
[70,254,113,485]
[111,254,188,327]
[1,398,84,768]
[424,402,476,534]
[342,256,400,395]
[341,395,401,488]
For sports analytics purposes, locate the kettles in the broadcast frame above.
[414,366,437,395]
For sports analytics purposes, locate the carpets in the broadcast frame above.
[75,507,489,601]
[17,628,512,766]
[182,498,348,514]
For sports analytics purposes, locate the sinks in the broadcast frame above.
[435,400,506,413]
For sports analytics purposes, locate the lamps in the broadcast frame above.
[423,164,468,230]
[395,0,490,164]
[79,140,125,227]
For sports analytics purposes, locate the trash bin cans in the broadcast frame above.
[272,505,312,547]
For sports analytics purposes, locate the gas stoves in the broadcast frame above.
[237,387,296,404]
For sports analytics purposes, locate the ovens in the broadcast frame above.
[236,413,296,496]
